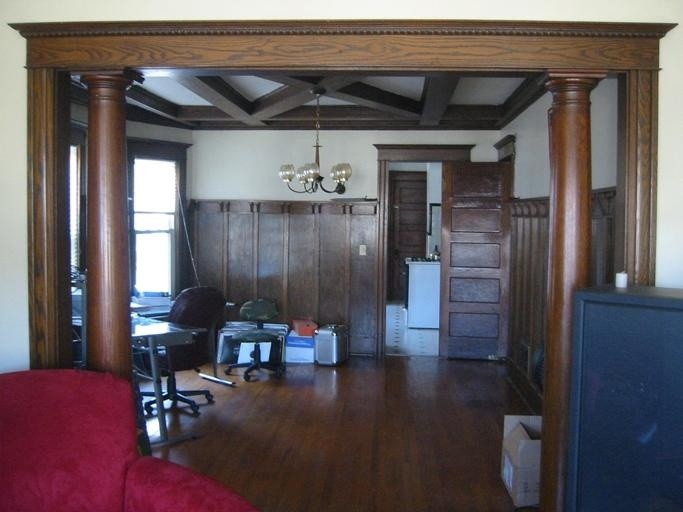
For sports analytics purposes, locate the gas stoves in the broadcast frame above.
[406,255,440,263]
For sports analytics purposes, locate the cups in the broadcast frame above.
[615,273,628,288]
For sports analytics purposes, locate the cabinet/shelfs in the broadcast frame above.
[565,285,683,512]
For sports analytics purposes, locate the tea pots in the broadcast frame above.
[430,245,440,261]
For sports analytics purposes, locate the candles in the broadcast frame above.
[616,270,628,289]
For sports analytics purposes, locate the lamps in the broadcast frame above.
[279,87,352,194]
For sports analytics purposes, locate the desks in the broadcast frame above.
[72,316,207,449]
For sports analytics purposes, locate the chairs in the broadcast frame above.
[132,286,224,417]
[0,370,261,512]
[225,298,285,381]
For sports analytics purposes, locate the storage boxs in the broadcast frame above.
[501,415,542,507]
[408,264,441,329]
[314,324,349,367]
